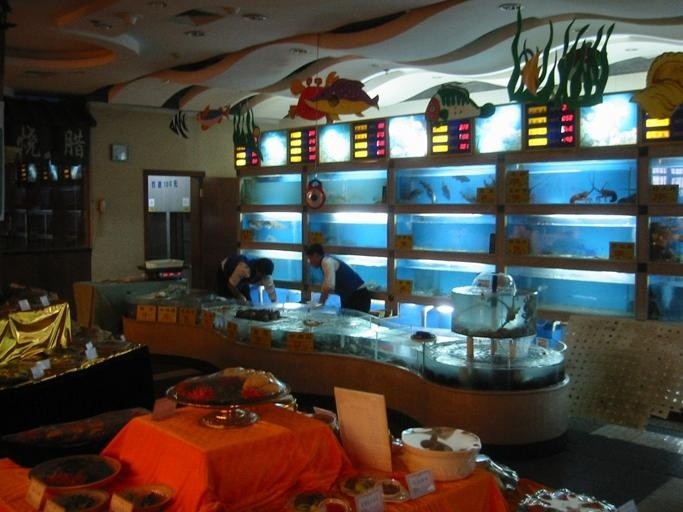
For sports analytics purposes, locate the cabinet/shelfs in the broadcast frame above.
[233,143,683,321]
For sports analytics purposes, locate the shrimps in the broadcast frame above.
[570,171,595,203]
[592,182,617,202]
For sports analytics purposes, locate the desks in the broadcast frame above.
[117,281,572,448]
[0,280,148,425]
[2,394,615,511]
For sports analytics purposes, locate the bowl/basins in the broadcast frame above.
[400,427,482,482]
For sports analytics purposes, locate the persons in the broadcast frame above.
[304,242,371,315]
[215,254,277,305]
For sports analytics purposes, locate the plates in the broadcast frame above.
[27,455,172,512]
[286,475,410,512]
[165,371,291,430]
